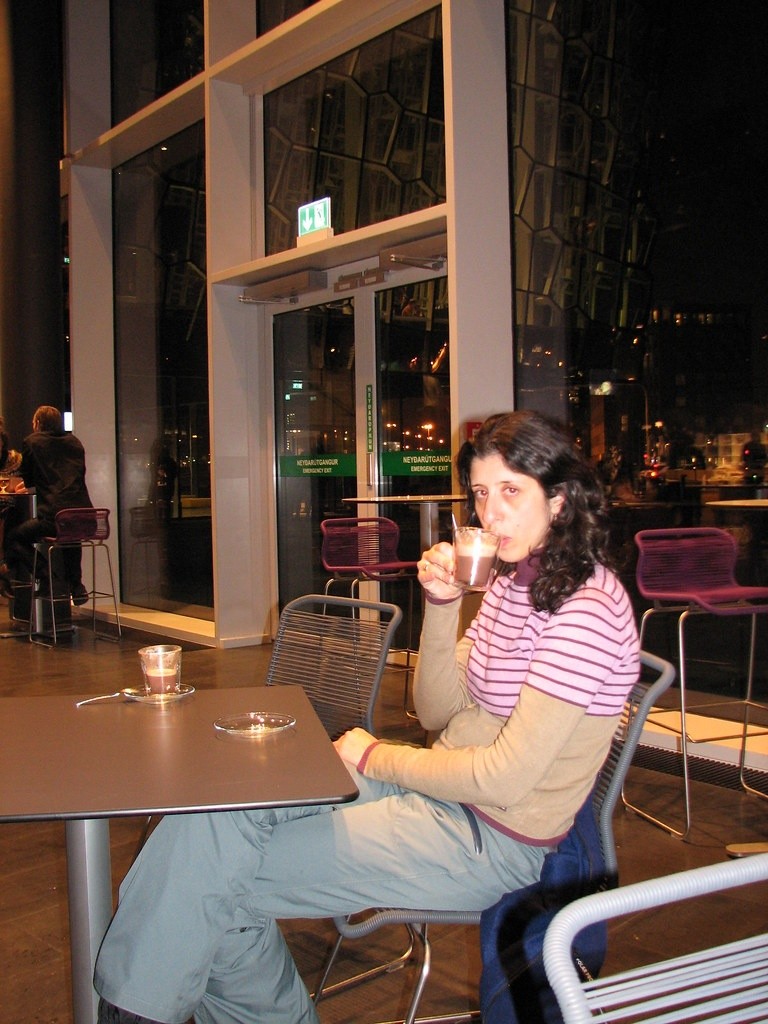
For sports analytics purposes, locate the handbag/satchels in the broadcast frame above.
[480,801,605,1023]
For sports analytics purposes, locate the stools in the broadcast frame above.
[127,506,164,601]
[313,516,420,720]
[28,508,123,648]
[619,526,768,840]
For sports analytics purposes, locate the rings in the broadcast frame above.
[424,562,431,570]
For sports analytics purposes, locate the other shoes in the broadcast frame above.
[0,586,15,599]
[69,579,89,605]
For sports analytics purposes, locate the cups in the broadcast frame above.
[450,527,503,591]
[138,645,182,696]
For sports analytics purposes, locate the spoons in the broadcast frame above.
[76,688,140,708]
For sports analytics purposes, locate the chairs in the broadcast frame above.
[542,851,767,1024]
[131,593,417,1000]
[312,649,677,1024]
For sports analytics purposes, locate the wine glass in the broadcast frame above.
[0,472,10,494]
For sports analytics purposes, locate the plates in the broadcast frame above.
[123,683,195,704]
[213,712,296,738]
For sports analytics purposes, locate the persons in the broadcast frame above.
[93,410,641,1024]
[8,406,94,606]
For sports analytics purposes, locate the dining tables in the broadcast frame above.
[1,492,76,638]
[704,500,768,857]
[341,494,469,619]
[0,685,359,1024]
